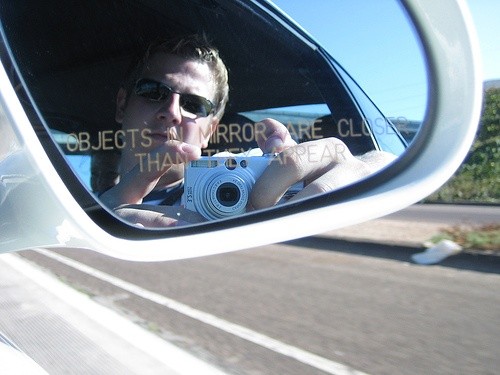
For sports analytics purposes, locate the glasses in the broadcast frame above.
[127,79,220,118]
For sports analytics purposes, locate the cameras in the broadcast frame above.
[180,152,304,223]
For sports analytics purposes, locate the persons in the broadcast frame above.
[97,30,400,241]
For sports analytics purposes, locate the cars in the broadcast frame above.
[0,1,409,230]
